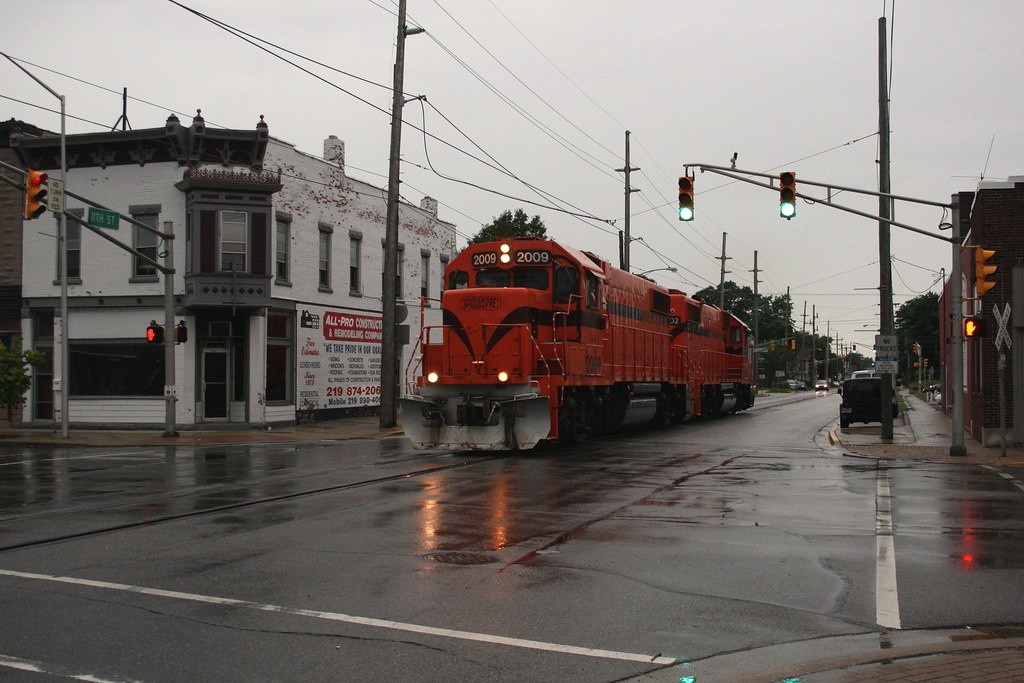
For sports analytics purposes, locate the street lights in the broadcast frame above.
[801,349,822,382]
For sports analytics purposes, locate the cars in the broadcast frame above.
[832,381,839,387]
[815,380,829,391]
[787,380,798,390]
[798,382,807,390]
[923,383,941,393]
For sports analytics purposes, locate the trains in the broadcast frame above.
[398,237,755,454]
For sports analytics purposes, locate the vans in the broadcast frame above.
[851,370,898,418]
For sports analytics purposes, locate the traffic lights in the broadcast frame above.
[175,327,188,343]
[913,344,916,353]
[975,246,998,299]
[678,177,694,221]
[147,326,164,344]
[26,169,47,219]
[924,359,928,367]
[780,171,796,217]
[965,319,986,336]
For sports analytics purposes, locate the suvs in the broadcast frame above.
[840,377,882,428]
